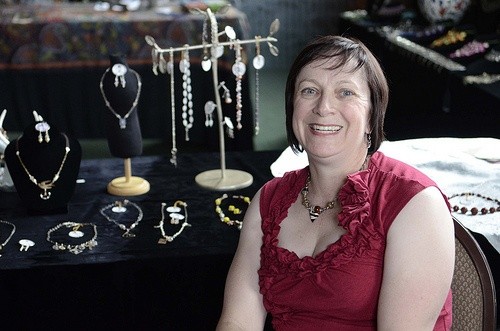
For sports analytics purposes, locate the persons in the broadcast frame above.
[215,35,455,331]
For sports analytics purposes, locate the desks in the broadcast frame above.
[0,137,500,331]
[0,0,255,151]
[335,9,500,137]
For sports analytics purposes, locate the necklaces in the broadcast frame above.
[167,36,265,169]
[215,194,252,230]
[0,219,16,258]
[46,221,98,255]
[448,192,500,215]
[301,154,368,223]
[98,66,142,129]
[15,130,71,200]
[152,201,192,252]
[100,199,143,238]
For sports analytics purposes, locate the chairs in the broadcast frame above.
[451,213,498,331]
